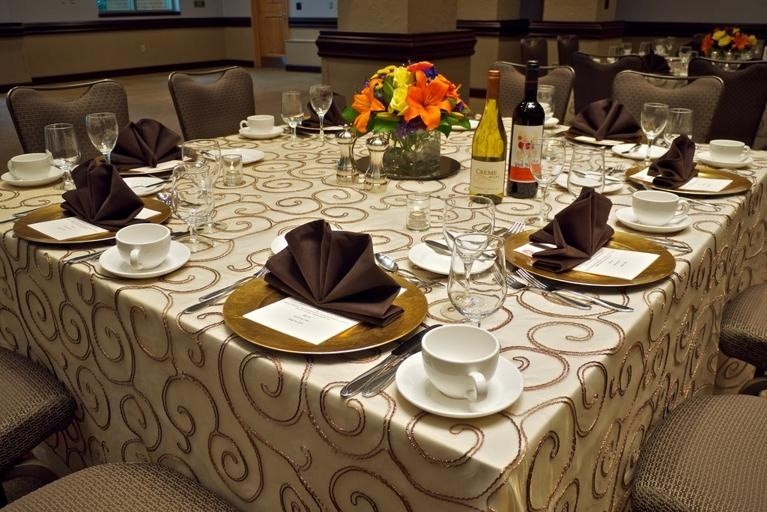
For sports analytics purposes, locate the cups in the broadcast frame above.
[6,152,51,179]
[421,322,501,402]
[114,223,172,270]
[221,154,243,186]
[240,114,275,133]
[710,139,751,161]
[633,190,689,225]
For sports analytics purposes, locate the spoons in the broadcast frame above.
[375,253,445,298]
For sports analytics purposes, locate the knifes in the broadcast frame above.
[363,344,424,398]
[425,239,484,261]
[340,324,442,398]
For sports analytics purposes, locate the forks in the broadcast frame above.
[503,271,634,312]
[501,220,525,241]
[183,265,271,314]
[517,268,591,310]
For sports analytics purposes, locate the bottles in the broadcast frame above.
[335,123,358,181]
[364,135,387,189]
[469,69,507,205]
[506,60,545,198]
[406,192,430,230]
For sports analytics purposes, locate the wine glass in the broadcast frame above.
[181,139,227,233]
[448,235,509,327]
[171,164,214,251]
[527,138,566,228]
[664,106,693,150]
[445,194,496,309]
[608,38,701,75]
[45,123,81,189]
[566,147,606,201]
[309,83,333,141]
[282,91,304,143]
[85,112,119,164]
[641,102,669,165]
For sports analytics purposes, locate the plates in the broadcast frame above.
[555,170,623,194]
[239,126,284,139]
[616,207,692,235]
[501,229,677,287]
[698,151,754,168]
[625,164,752,196]
[298,121,350,131]
[1,166,64,187]
[612,142,669,159]
[396,349,523,420]
[201,147,265,165]
[408,239,497,276]
[13,196,170,244]
[355,149,461,179]
[100,240,191,278]
[122,176,166,198]
[223,268,430,354]
